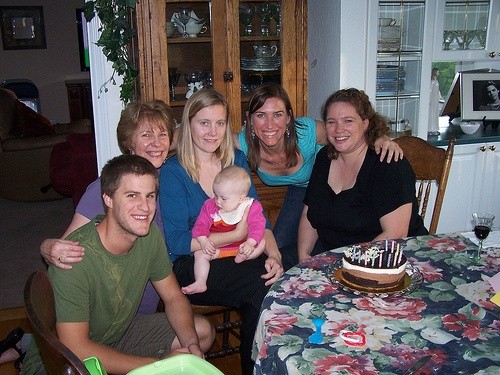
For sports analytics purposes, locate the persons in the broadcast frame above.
[428,68,440,135]
[48,155,217,375]
[475,81,500,111]
[159,88,285,375]
[171,83,405,249]
[279,88,430,271]
[180,166,266,294]
[0,100,170,375]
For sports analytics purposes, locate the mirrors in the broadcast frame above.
[431,60,500,138]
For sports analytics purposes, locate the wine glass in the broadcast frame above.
[470,213,496,265]
[237,0,281,37]
[443,30,486,52]
[174,6,192,38]
[168,66,213,102]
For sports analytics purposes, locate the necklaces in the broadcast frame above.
[487,100,500,110]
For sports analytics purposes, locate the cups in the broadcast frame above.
[176,23,207,38]
[379,17,397,27]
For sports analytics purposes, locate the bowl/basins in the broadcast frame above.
[460,122,480,135]
[166,21,175,39]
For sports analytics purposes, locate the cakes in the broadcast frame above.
[341,240,407,289]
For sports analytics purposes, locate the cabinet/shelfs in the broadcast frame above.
[364,0,500,142]
[413,141,500,236]
[123,0,307,230]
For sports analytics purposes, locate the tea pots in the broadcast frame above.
[253,45,277,56]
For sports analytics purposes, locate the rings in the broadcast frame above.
[58,257,63,263]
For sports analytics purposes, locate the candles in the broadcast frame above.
[351,236,404,267]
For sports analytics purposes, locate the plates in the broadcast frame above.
[240,56,282,70]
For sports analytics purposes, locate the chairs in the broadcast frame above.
[188,171,290,361]
[391,134,455,236]
[23,270,91,375]
[0,86,92,202]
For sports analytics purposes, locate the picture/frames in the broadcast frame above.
[0,6,47,50]
[458,71,500,127]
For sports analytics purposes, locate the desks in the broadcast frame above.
[250,226,500,375]
